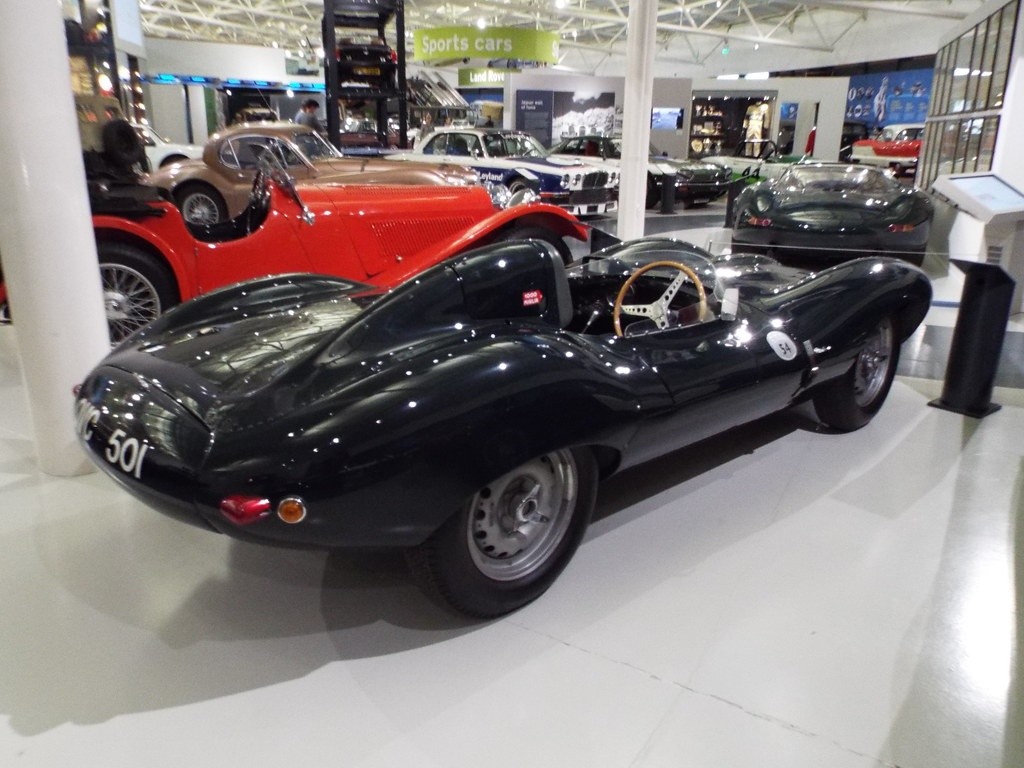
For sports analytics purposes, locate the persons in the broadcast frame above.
[743,101,769,157]
[295,98,324,132]
[485,116,494,128]
[420,115,434,142]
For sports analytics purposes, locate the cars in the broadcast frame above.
[701,140,823,188]
[150,121,480,234]
[332,33,399,91]
[386,128,612,219]
[0,158,592,348]
[545,133,730,206]
[71,234,935,620]
[848,124,925,174]
[727,166,937,268]
